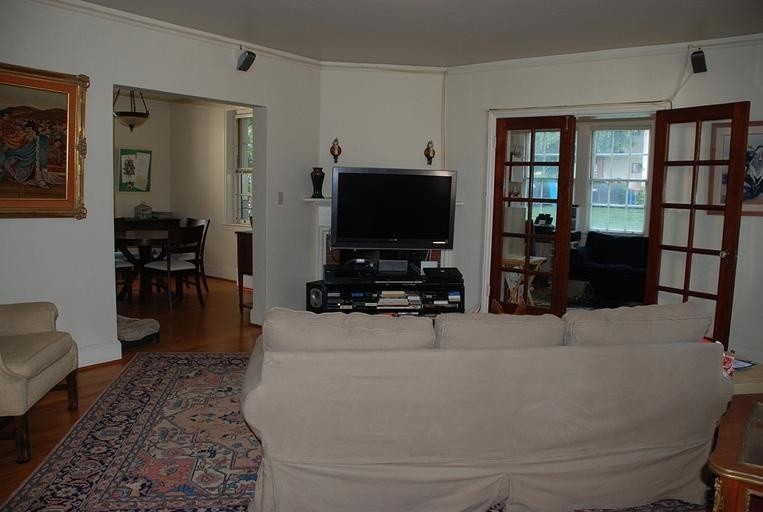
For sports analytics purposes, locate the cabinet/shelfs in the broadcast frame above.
[502,206,527,302]
[320,285,463,316]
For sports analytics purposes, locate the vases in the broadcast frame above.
[310,166,324,198]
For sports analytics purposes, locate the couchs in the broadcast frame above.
[238,303,732,512]
[564,231,649,300]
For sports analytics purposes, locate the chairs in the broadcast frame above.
[1,301,78,468]
[112,209,210,314]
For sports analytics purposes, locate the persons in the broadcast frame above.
[0,113,65,189]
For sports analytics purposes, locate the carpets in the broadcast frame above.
[1,349,260,510]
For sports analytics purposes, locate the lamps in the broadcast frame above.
[112,88,150,130]
[424,139,435,164]
[329,135,342,163]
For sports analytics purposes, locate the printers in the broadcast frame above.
[534,214,554,235]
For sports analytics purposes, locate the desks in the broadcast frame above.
[708,393,762,512]
[526,228,581,289]
[233,229,251,312]
[500,253,546,305]
[717,350,761,404]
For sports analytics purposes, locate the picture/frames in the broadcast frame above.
[0,60,91,219]
[706,120,762,218]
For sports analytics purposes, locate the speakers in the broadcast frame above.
[306,280,323,313]
[237,51,256,71]
[691,51,707,73]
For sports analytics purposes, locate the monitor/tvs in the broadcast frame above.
[331,167,457,249]
